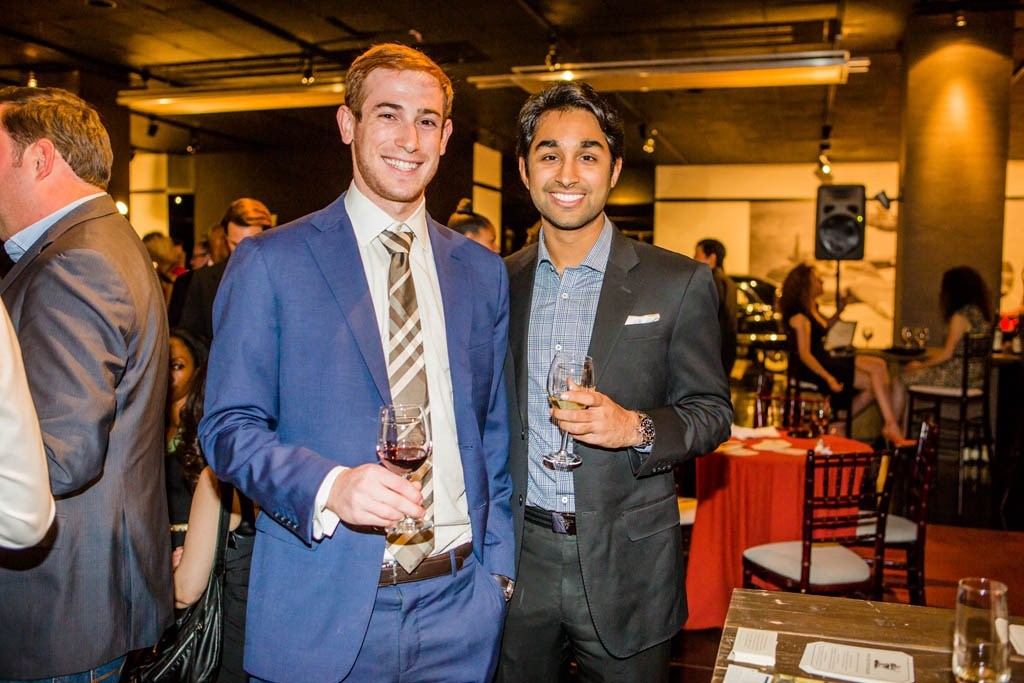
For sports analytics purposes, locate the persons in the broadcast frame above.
[143,198,273,683]
[690,238,737,358]
[890,265,999,436]
[449,197,502,254]
[1,86,169,683]
[488,80,733,682]
[778,263,917,446]
[196,42,516,683]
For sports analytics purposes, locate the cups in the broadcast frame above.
[952,577,1010,683]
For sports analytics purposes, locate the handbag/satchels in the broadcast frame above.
[121,476,233,683]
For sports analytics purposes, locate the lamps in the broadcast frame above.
[300,57,315,87]
[818,155,831,174]
[545,43,560,72]
[642,128,659,155]
[148,116,160,136]
[27,71,40,90]
[874,190,904,210]
[185,129,200,154]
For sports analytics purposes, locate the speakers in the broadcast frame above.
[816,184,866,260]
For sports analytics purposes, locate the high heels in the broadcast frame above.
[882,425,917,451]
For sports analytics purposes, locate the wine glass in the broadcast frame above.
[543,353,596,466]
[376,404,435,534]
[811,399,830,453]
[902,327,930,348]
[862,327,874,348]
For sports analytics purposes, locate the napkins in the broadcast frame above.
[731,424,781,440]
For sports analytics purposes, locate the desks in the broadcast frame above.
[680,427,876,632]
[984,350,1024,530]
[711,588,1024,683]
[848,341,940,438]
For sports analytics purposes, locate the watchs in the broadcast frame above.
[489,572,514,601]
[634,411,654,449]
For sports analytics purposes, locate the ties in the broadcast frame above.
[377,230,434,573]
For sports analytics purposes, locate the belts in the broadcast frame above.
[378,539,473,586]
[521,504,576,536]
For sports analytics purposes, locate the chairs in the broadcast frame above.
[742,325,995,608]
